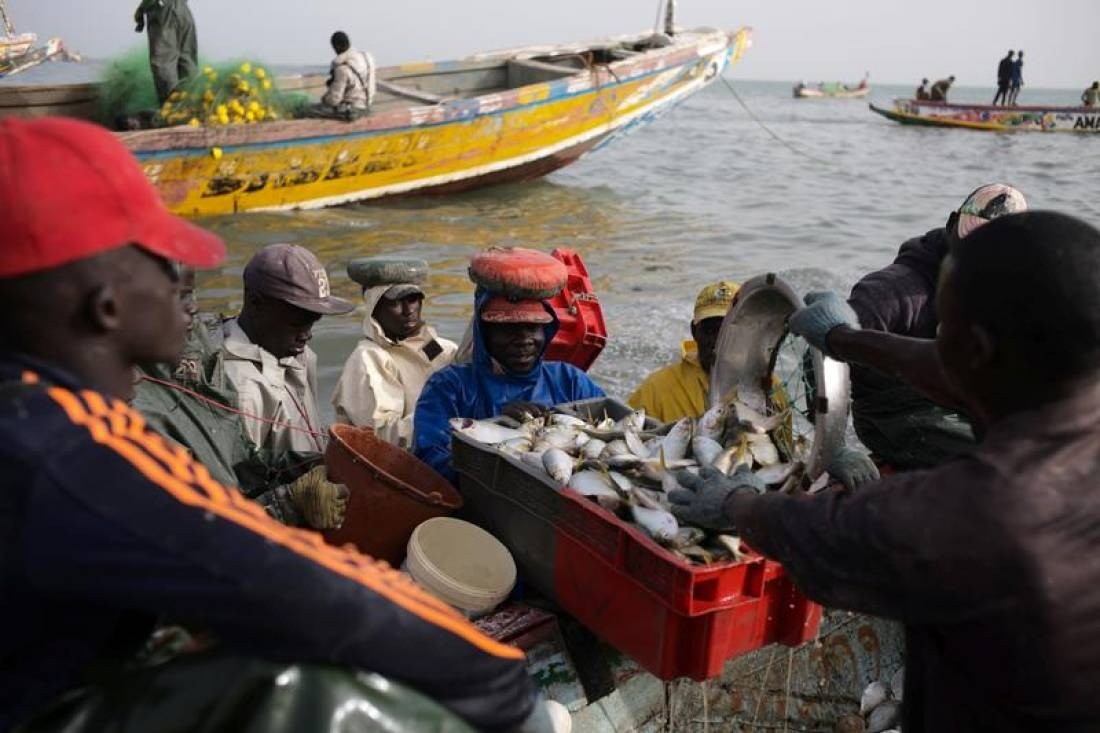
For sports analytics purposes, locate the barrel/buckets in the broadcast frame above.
[324,421,463,570]
[400,515,515,621]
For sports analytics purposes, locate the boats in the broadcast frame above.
[0,1,754,224]
[794,84,868,99]
[871,95,1100,134]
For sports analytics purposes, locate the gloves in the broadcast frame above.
[662,462,764,529]
[790,287,865,355]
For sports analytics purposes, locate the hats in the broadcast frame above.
[0,108,227,278]
[379,282,427,300]
[952,183,1030,240]
[691,280,745,324]
[480,291,554,325]
[245,241,356,314]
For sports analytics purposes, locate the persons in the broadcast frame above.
[0,115,573,733]
[130,262,349,529]
[134,0,198,110]
[222,245,357,453]
[332,257,459,452]
[294,32,376,120]
[667,210,1100,733]
[917,75,955,101]
[412,247,606,491]
[1081,82,1100,107]
[628,281,880,496]
[846,183,1029,470]
[993,51,1023,106]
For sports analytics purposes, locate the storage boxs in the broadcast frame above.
[543,246,607,372]
[455,397,822,683]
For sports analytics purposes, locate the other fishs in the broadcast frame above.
[448,405,806,571]
